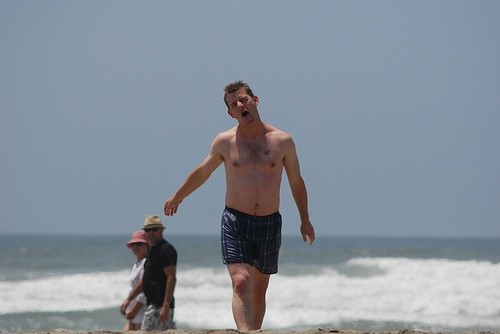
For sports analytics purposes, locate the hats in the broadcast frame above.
[142,215,166,230]
[127,230,151,248]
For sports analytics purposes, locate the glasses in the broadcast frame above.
[131,243,147,247]
[144,228,159,232]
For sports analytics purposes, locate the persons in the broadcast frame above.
[164,80,315,332]
[120,216,178,331]
[125,230,149,331]
